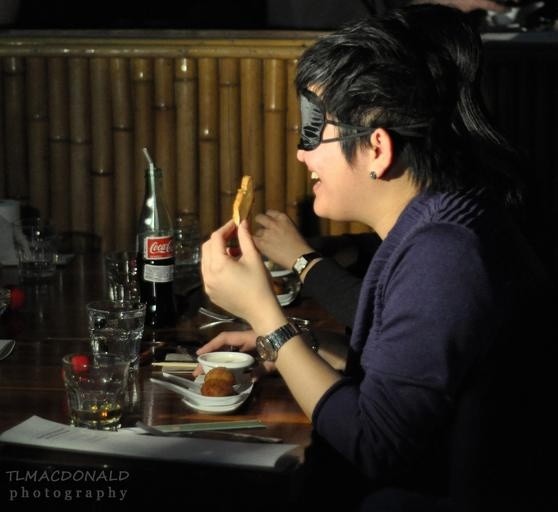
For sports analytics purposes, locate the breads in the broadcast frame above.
[233,175,255,227]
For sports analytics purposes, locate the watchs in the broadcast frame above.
[292,251,323,277]
[255,321,319,363]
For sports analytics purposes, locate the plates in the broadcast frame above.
[263,258,301,305]
[179,377,254,412]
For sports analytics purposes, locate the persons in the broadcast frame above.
[200,23,546,512]
[250,2,558,344]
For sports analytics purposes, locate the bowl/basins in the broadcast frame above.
[196,349,255,375]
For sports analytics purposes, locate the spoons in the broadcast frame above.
[149,371,244,407]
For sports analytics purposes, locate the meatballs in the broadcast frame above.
[205,367,236,386]
[201,378,234,396]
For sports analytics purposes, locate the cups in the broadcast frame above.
[60,353,131,432]
[85,249,147,373]
[12,216,57,277]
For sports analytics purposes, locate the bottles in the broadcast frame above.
[174,213,200,266]
[137,168,176,326]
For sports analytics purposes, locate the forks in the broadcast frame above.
[197,305,312,324]
[195,316,231,330]
[135,416,283,450]
